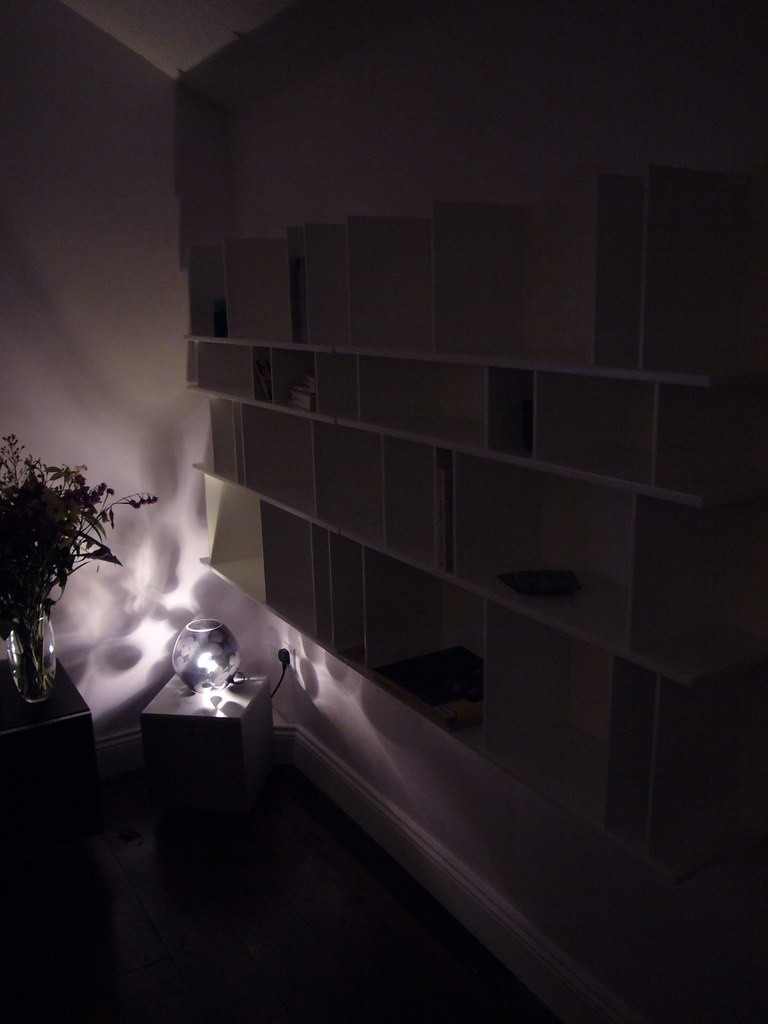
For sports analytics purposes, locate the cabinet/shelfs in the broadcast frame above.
[185,162,768,884]
[0,648,103,864]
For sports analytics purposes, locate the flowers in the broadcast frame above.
[0,433,158,696]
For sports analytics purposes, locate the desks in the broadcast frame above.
[139,672,275,820]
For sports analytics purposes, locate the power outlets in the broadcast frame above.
[281,641,296,674]
